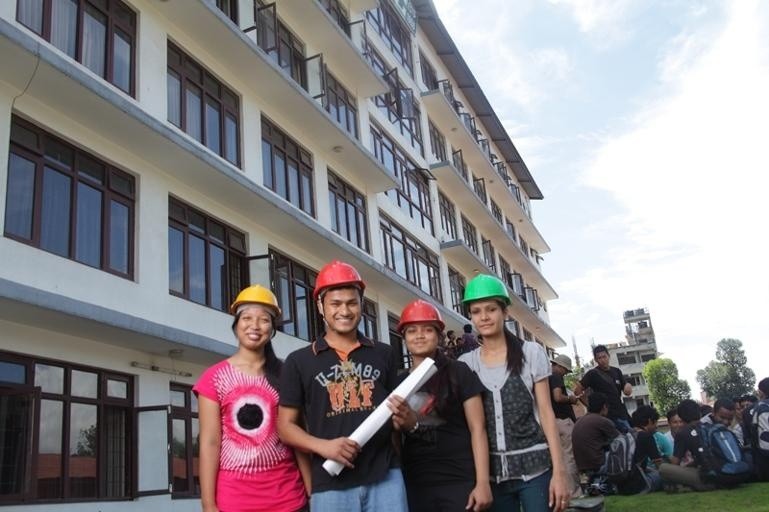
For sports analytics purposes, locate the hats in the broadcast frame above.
[550,355,572,372]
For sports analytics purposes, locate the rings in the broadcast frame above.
[561,500,568,504]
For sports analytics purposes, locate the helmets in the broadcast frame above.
[397,301,445,332]
[313,261,365,301]
[230,285,281,318]
[462,274,512,305]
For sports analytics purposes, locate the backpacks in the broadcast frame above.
[605,428,644,477]
[698,422,751,480]
[752,401,769,457]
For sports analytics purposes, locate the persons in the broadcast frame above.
[396,299,495,512]
[571,377,769,496]
[456,273,570,512]
[550,354,580,499]
[438,324,483,359]
[574,345,632,431]
[189,284,313,511]
[276,260,419,512]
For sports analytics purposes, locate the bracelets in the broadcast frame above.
[409,422,418,435]
